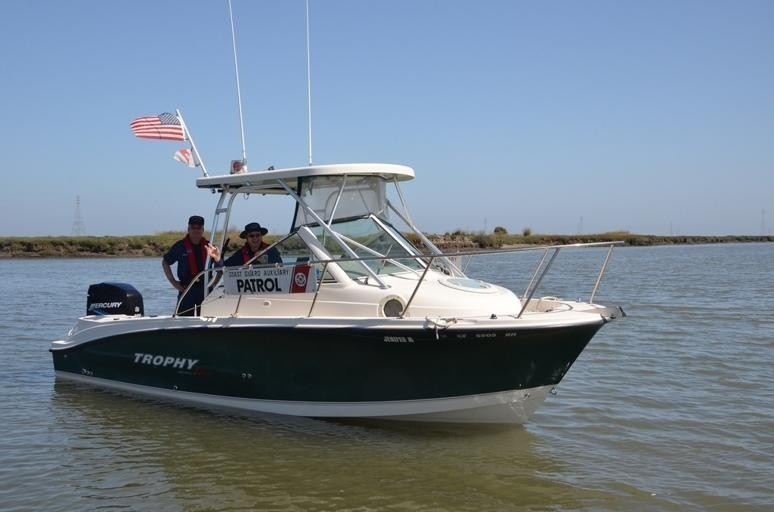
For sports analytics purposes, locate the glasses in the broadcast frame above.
[245,233,261,237]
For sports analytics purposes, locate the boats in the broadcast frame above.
[46,2,629,428]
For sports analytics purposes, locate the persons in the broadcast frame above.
[205,223,284,279]
[161,215,223,316]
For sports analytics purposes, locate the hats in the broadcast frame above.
[189,215,205,224]
[240,222,268,238]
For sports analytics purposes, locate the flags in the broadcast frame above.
[129,112,185,140]
